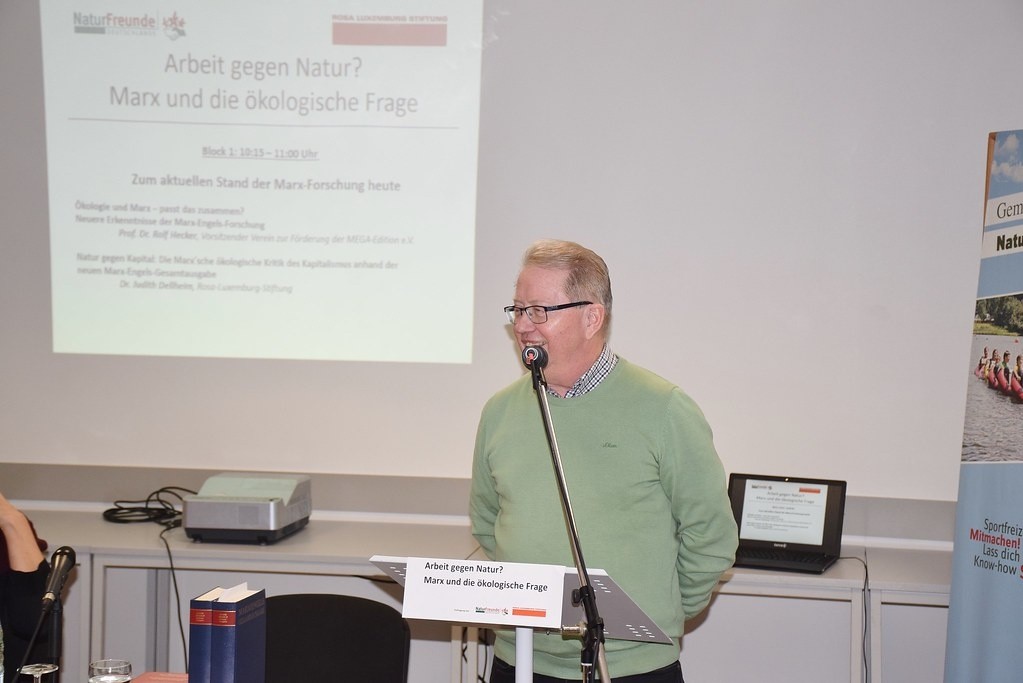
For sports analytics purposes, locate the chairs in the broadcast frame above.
[267,593,410,683]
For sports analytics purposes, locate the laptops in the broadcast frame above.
[727,473,847,575]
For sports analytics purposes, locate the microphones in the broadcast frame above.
[41,546,76,612]
[521,345,548,370]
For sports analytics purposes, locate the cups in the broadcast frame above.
[88,660,132,683]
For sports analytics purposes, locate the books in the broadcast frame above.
[189,583,265,683]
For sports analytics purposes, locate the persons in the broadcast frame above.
[468,241,738,683]
[979,347,1023,391]
[0,493,61,683]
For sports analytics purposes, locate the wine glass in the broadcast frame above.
[17,664,59,683]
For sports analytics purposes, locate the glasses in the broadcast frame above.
[504,302,593,325]
